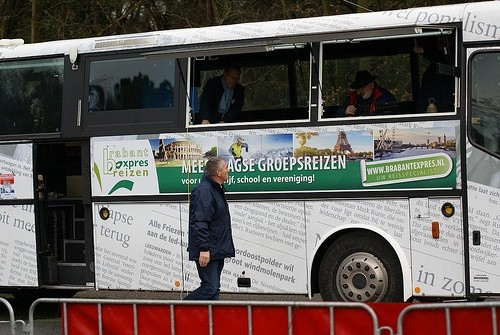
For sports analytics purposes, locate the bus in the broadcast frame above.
[0,1,500,335]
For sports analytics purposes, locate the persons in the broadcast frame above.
[413,36,455,112]
[229,137,248,165]
[192,63,244,125]
[182,157,235,301]
[338,70,397,117]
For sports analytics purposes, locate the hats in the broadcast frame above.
[349,70,376,90]
